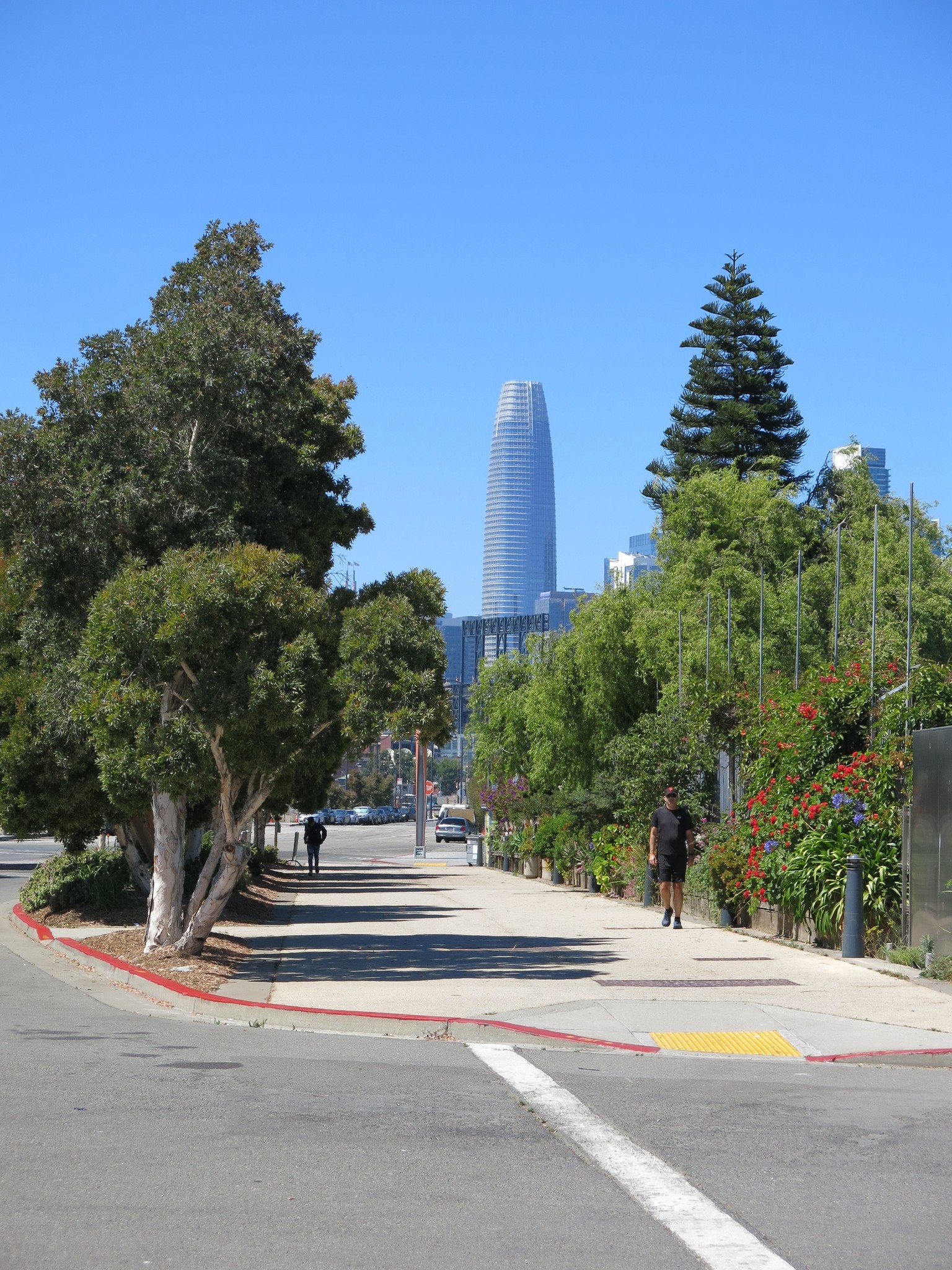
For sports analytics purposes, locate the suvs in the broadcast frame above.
[435,803,476,825]
[353,806,373,825]
[372,806,395,825]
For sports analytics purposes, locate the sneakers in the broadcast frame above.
[662,908,673,927]
[673,919,682,929]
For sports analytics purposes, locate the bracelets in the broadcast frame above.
[689,852,694,856]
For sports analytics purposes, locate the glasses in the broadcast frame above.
[666,794,677,798]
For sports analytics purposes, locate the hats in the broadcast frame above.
[308,817,314,823]
[664,787,678,796]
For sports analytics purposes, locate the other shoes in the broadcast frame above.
[315,867,319,873]
[308,870,313,876]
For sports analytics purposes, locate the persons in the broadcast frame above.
[303,816,327,876]
[649,787,694,929]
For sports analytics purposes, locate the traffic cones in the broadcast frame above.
[270,815,273,822]
[295,815,298,822]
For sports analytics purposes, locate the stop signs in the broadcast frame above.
[425,781,434,795]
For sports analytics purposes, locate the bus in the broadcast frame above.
[402,794,416,808]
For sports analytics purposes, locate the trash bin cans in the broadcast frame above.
[465,833,483,867]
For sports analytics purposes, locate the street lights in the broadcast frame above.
[375,732,381,774]
[345,746,362,791]
[442,677,491,801]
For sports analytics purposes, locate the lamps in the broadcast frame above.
[844,852,867,869]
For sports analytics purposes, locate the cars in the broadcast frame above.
[426,802,442,811]
[400,808,416,822]
[332,809,351,825]
[298,810,324,826]
[393,808,403,822]
[346,810,359,825]
[321,808,336,825]
[435,817,479,844]
